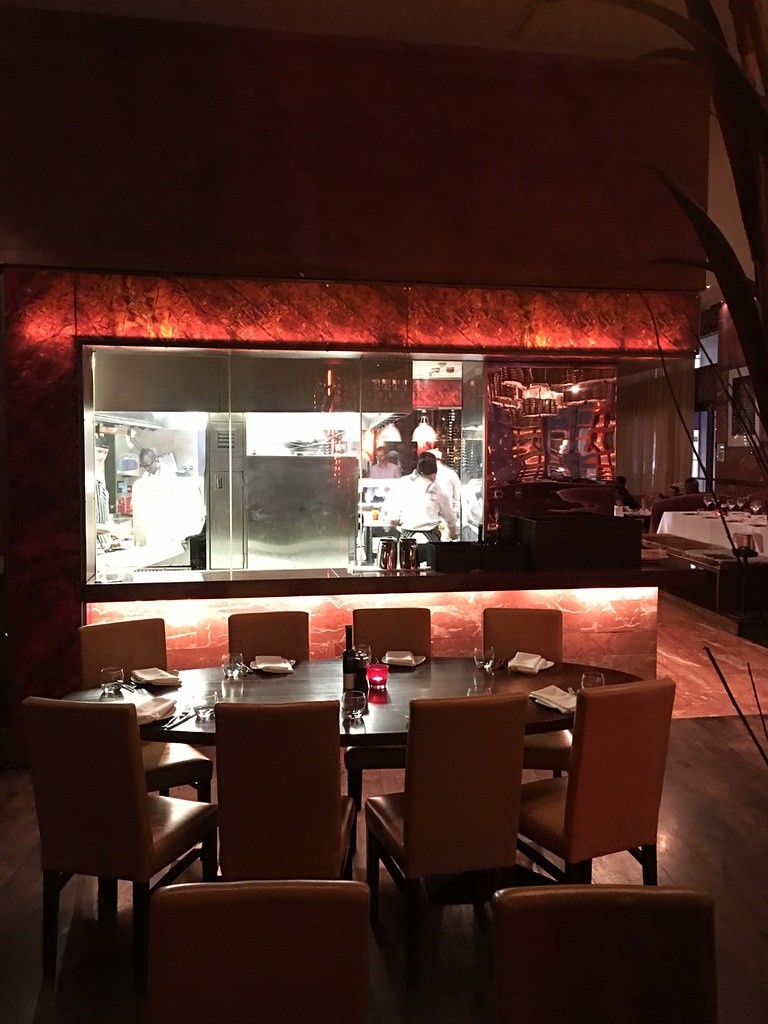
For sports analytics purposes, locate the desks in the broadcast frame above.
[64,655,646,851]
[656,509,768,558]
[626,509,652,526]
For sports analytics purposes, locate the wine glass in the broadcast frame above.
[703,495,762,520]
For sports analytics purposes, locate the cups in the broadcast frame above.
[222,652,244,678]
[355,657,370,691]
[372,507,379,520]
[614,506,623,516]
[340,691,366,721]
[581,672,606,689]
[100,667,124,695]
[473,647,495,668]
[354,645,372,664]
[377,539,397,569]
[367,664,389,691]
[191,689,217,721]
[400,539,419,569]
[467,687,492,697]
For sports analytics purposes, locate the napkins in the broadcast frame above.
[128,666,183,688]
[528,684,577,714]
[134,700,176,726]
[256,655,295,675]
[385,651,416,667]
[508,651,546,675]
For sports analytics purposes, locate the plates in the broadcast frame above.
[250,660,296,669]
[158,706,176,720]
[130,669,179,684]
[381,656,426,665]
[507,658,555,669]
[684,511,767,526]
[534,699,557,709]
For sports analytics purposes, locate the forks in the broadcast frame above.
[164,706,191,730]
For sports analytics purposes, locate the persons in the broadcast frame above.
[399,457,458,569]
[368,446,403,478]
[684,478,699,494]
[615,476,641,511]
[95,436,133,549]
[427,449,460,542]
[377,452,435,541]
[139,449,162,477]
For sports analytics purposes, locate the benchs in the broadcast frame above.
[642,533,768,609]
[649,492,714,533]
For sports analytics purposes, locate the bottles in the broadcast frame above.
[343,624,356,691]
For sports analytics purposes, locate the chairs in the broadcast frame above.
[24,604,719,1024]
